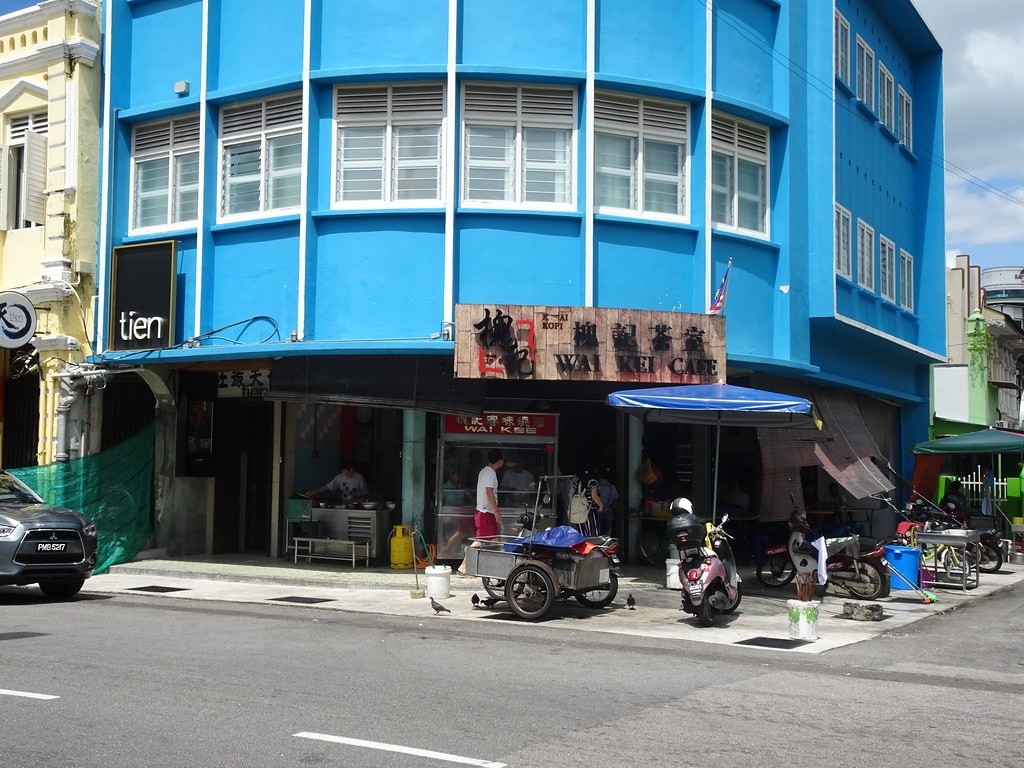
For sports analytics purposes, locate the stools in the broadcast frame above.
[821,520,869,538]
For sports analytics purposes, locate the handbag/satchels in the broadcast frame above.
[638,459,661,485]
[811,536,828,585]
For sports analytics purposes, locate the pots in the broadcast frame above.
[1007,552,1024,565]
[362,502,377,509]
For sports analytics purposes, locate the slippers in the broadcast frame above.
[455,571,473,577]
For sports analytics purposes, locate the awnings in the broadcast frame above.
[987,380,1024,390]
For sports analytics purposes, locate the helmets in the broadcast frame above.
[670,498,692,514]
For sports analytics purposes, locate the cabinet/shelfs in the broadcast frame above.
[292,535,372,570]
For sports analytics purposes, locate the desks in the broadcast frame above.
[915,530,979,592]
[633,515,712,557]
[806,507,880,536]
[717,514,761,550]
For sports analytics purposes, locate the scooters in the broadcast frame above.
[668,512,744,626]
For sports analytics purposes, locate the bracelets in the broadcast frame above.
[495,510,499,512]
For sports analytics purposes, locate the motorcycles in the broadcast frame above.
[756,494,888,600]
[916,500,1006,574]
[465,492,620,620]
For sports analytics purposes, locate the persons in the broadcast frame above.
[570,479,603,536]
[816,483,847,528]
[597,468,618,535]
[444,466,472,506]
[458,449,504,575]
[500,455,535,508]
[440,518,474,555]
[304,463,368,505]
[803,479,818,510]
[945,480,966,511]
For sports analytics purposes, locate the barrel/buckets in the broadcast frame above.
[665,543,684,589]
[885,545,922,590]
[1012,517,1024,524]
[425,566,451,599]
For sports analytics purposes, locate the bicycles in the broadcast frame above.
[873,521,982,576]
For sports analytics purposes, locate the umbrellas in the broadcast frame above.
[910,428,1024,517]
[607,383,816,526]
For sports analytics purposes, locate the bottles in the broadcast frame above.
[648,490,653,501]
[644,499,668,517]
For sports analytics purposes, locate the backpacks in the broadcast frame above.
[567,479,596,524]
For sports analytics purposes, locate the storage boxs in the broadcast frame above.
[667,514,708,550]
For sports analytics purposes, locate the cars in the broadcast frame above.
[0,469,98,600]
